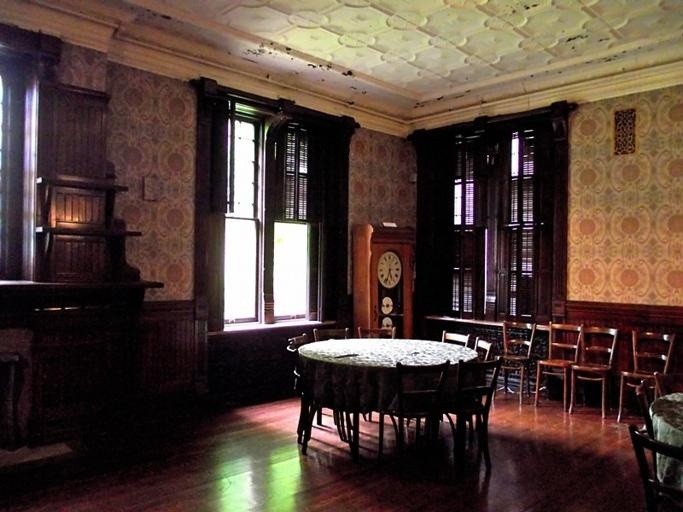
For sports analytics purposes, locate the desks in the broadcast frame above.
[2,277,165,501]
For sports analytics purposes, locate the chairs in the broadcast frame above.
[494,320,537,405]
[630,370,683,509]
[617,329,676,422]
[569,326,619,418]
[533,321,583,412]
[284,326,503,472]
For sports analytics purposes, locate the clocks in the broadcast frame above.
[377,251,403,289]
